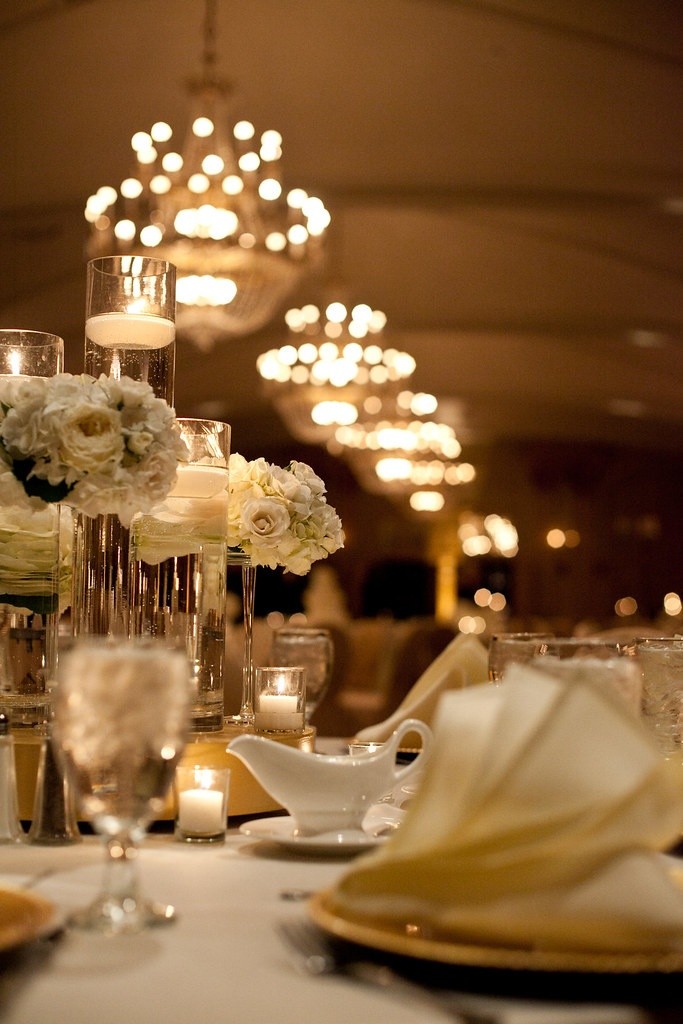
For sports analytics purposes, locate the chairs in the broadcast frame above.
[312,623,452,737]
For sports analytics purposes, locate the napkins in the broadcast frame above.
[323,657,683,954]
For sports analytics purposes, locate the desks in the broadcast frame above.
[0,734,683,1024]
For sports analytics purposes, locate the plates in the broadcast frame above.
[238,815,396,856]
[0,885,68,953]
[308,857,683,973]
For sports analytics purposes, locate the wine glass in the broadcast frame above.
[43,633,197,934]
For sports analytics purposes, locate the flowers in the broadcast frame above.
[0,371,346,611]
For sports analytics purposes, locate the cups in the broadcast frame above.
[254,667,307,738]
[273,628,335,725]
[489,633,683,753]
[83,255,177,408]
[77,418,229,743]
[0,328,64,734]
[173,764,232,846]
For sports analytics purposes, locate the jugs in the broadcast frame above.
[225,718,434,836]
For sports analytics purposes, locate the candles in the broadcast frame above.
[180,790,224,832]
[260,696,297,729]
